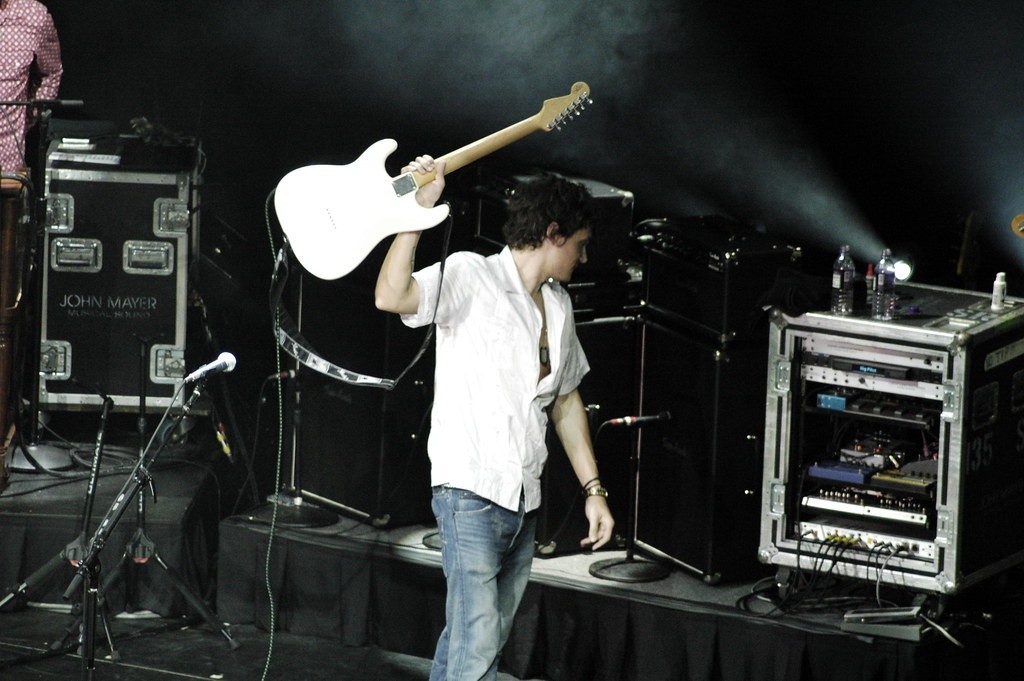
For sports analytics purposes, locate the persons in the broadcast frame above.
[0,0,64,176]
[376,155,615,681]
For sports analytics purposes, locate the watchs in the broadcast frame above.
[586,488,608,497]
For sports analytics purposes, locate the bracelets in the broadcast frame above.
[582,478,600,490]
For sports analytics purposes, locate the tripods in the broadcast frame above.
[0,333,246,664]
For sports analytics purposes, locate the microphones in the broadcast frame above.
[268,368,300,383]
[183,352,236,383]
[609,411,671,427]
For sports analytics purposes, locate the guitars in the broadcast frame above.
[273,81,596,282]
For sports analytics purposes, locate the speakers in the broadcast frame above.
[533,314,778,585]
[292,240,443,531]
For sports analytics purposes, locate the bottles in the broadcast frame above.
[865,265,874,304]
[831,246,855,316]
[872,249,895,320]
[991,272,1007,311]
